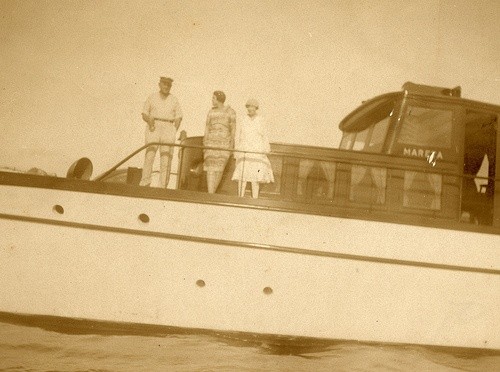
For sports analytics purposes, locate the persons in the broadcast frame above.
[231,99,276,199]
[202,91,236,194]
[138,76,183,189]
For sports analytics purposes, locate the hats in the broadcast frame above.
[245,99,259,109]
[160,76,174,84]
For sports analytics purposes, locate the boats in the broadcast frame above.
[1,82,500,353]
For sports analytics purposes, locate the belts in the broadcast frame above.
[153,118,173,123]
